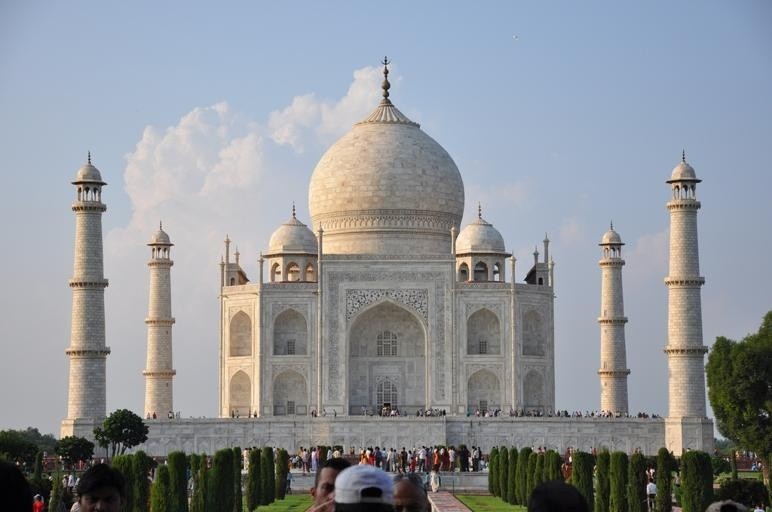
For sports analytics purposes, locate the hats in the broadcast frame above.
[334,465,393,504]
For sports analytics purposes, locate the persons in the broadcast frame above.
[207,456,213,469]
[146,412,157,420]
[249,410,257,417]
[530,444,772,512]
[368,407,408,417]
[415,409,446,417]
[13,453,195,512]
[168,411,172,420]
[465,408,661,418]
[304,457,432,512]
[232,410,239,418]
[311,409,336,417]
[244,444,495,493]
